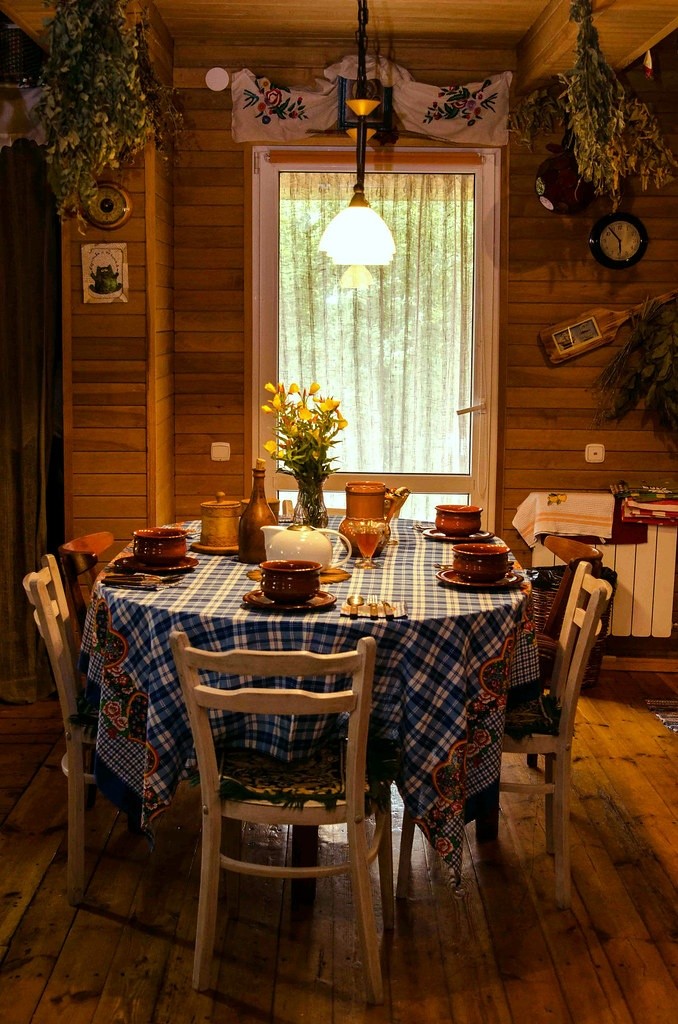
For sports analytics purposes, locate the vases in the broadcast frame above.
[292,475,329,529]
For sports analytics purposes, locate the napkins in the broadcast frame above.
[338,600,407,617]
[119,572,171,591]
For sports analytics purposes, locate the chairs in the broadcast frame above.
[58,529,115,639]
[168,629,393,1007]
[392,558,614,913]
[24,553,103,908]
[525,532,603,769]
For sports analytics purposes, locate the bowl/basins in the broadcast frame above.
[435,505,483,537]
[131,528,187,567]
[258,559,323,605]
[450,542,511,584]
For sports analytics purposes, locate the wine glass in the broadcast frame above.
[353,526,381,570]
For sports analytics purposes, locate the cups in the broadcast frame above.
[200,491,241,546]
[241,498,279,523]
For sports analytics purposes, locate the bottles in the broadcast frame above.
[236,457,278,564]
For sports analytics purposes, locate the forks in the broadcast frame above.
[366,595,378,621]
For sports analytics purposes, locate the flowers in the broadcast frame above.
[262,379,348,526]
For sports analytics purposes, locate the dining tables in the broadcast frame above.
[75,512,538,922]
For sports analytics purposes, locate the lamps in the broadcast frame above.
[318,0,398,265]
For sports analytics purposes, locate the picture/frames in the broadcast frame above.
[339,72,393,130]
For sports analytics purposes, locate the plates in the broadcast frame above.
[192,541,239,555]
[243,590,337,613]
[113,556,199,575]
[422,528,495,542]
[435,568,524,589]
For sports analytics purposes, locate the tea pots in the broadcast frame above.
[260,506,352,572]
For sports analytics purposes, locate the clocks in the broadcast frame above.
[588,211,649,270]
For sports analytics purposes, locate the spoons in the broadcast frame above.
[346,596,364,620]
[104,575,185,584]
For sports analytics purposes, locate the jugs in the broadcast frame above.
[339,481,395,558]
